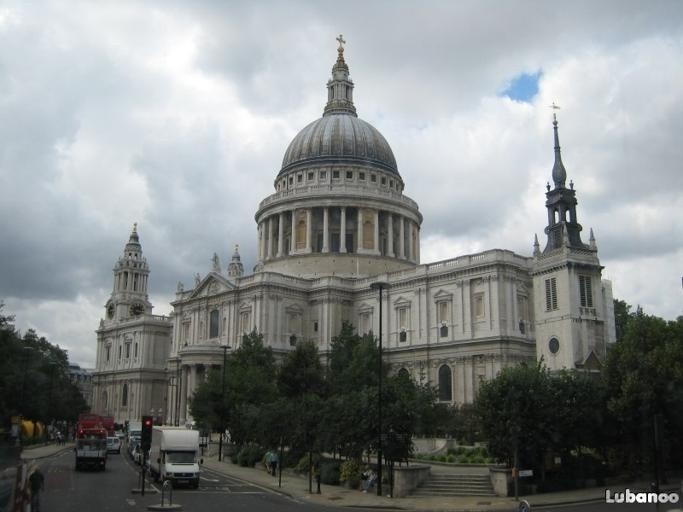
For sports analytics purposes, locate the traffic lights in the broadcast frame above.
[139,415,153,450]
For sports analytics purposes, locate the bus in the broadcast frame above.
[76,413,102,430]
[100,415,113,437]
[185,419,209,448]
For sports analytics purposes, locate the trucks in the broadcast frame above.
[0,406,45,512]
[74,427,108,471]
[146,424,201,488]
[126,418,142,443]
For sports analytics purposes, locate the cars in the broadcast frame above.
[127,437,143,465]
[112,430,124,438]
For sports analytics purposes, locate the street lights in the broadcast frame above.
[365,275,392,496]
[42,359,57,424]
[20,345,33,417]
[215,343,232,461]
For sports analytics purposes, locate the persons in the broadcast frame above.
[55,430,66,447]
[263,449,272,473]
[28,466,45,512]
[270,449,278,476]
[362,464,378,493]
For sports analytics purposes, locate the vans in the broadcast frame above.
[106,437,122,453]
